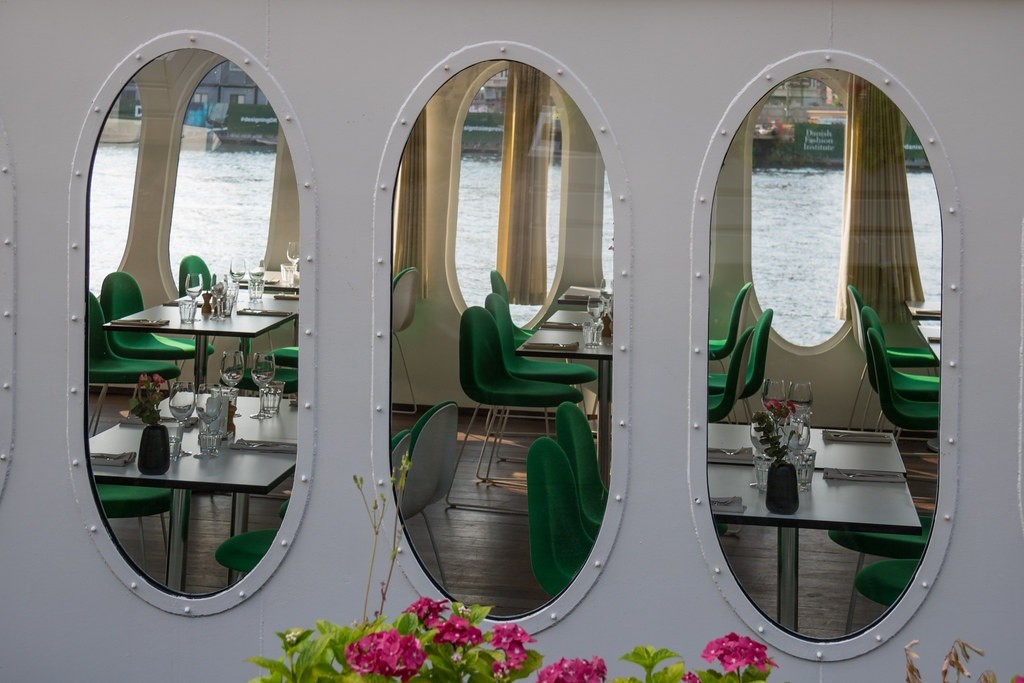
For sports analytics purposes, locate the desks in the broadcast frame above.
[905,301,941,322]
[102,305,300,397]
[177,289,299,347]
[915,325,941,361]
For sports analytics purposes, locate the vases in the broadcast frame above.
[765,458,800,514]
[137,424,171,476]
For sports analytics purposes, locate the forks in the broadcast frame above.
[117,318,161,324]
[241,438,297,448]
[90,451,126,460]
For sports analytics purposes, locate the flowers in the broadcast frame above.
[126,371,169,424]
[751,398,797,459]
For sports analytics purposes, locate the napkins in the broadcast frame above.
[90,450,137,468]
[237,306,294,318]
[231,437,296,456]
[823,466,906,484]
[162,297,212,307]
[118,408,197,428]
[274,293,300,299]
[542,321,583,330]
[821,428,893,444]
[232,277,280,284]
[110,319,170,327]
[708,446,753,461]
[711,495,747,512]
[523,341,580,351]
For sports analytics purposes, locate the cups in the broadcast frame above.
[227,283,241,303]
[248,279,265,301]
[210,387,240,406]
[785,448,816,493]
[179,300,198,324]
[280,263,297,286]
[582,322,604,349]
[217,294,235,316]
[753,455,775,496]
[198,417,221,455]
[162,421,184,462]
[259,381,286,415]
[586,295,605,322]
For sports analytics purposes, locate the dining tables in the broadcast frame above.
[708,422,908,474]
[539,309,591,331]
[514,325,612,480]
[237,268,300,290]
[557,285,601,307]
[87,390,297,592]
[708,463,920,630]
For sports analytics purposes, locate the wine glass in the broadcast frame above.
[185,273,204,321]
[194,383,223,459]
[599,279,614,315]
[169,381,196,458]
[287,241,299,286]
[209,273,228,321]
[606,299,613,346]
[249,260,265,304]
[220,350,245,418]
[250,352,275,420]
[749,378,815,489]
[230,257,246,303]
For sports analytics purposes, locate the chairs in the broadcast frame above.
[83,254,939,635]
